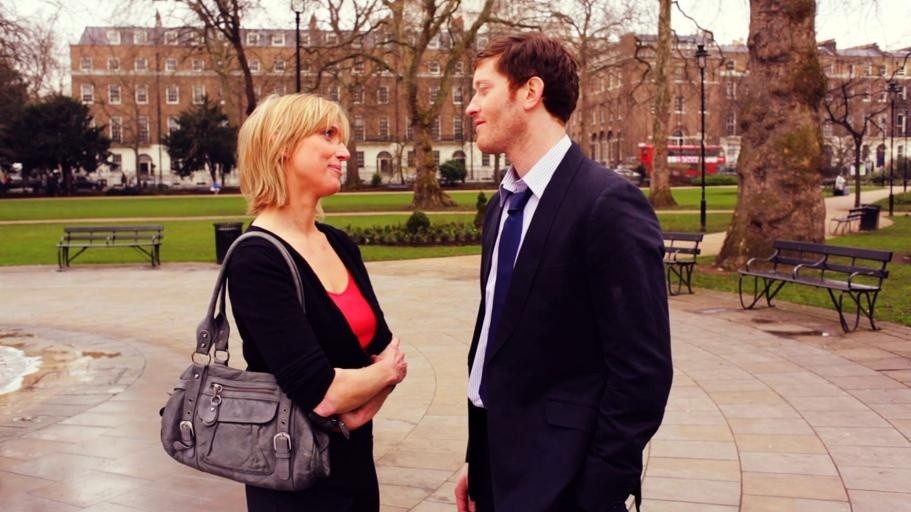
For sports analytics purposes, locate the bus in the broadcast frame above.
[638,142,726,178]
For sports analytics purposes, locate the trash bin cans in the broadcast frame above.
[214,222,244,264]
[834,177,846,195]
[861,205,882,230]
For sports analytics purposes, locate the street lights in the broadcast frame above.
[291,0,304,93]
[693,45,710,231]
[886,84,903,216]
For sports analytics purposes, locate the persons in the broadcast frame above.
[226,91,412,511]
[444,28,674,512]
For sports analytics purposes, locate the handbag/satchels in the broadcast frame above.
[160,363,333,493]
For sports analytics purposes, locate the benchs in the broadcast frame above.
[736,240,893,334]
[829,205,866,237]
[198,186,211,194]
[56,226,164,271]
[661,231,703,296]
[388,185,409,191]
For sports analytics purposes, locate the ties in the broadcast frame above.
[477,189,531,411]
